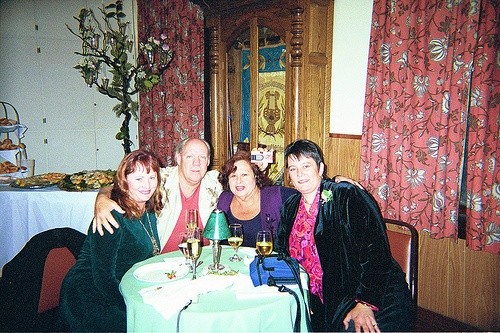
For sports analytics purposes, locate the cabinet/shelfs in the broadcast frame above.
[0,101,24,177]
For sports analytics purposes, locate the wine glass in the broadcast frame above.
[178,209,203,281]
[227,224,243,262]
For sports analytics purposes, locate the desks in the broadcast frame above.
[0,180,100,274]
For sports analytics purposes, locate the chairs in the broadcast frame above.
[383,218,419,305]
[0,227,87,333]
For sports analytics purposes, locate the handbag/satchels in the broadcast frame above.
[250,253,299,287]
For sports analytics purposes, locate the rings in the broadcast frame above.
[373,323,379,327]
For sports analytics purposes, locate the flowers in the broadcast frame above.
[321,189,333,206]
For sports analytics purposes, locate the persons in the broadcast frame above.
[91,137,223,253]
[274,139,414,333]
[58,149,170,333]
[217,151,364,248]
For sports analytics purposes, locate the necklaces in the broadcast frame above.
[138,212,160,256]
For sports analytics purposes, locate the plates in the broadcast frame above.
[133,262,190,282]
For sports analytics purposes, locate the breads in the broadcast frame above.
[0,139,26,150]
[0,117,18,126]
[0,161,25,174]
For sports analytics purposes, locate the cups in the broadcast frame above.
[256,231,273,255]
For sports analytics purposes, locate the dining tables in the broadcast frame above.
[119,245,311,333]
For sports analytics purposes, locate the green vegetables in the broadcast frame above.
[62,169,116,188]
[10,178,52,187]
